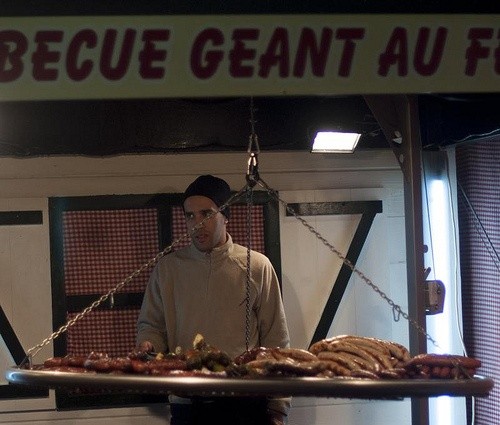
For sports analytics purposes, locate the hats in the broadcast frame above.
[184,175,231,219]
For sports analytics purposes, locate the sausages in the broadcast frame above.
[407,354,483,378]
[309,335,410,380]
[237,346,327,377]
[43,339,237,376]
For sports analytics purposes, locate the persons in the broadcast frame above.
[135,174,293,425]
